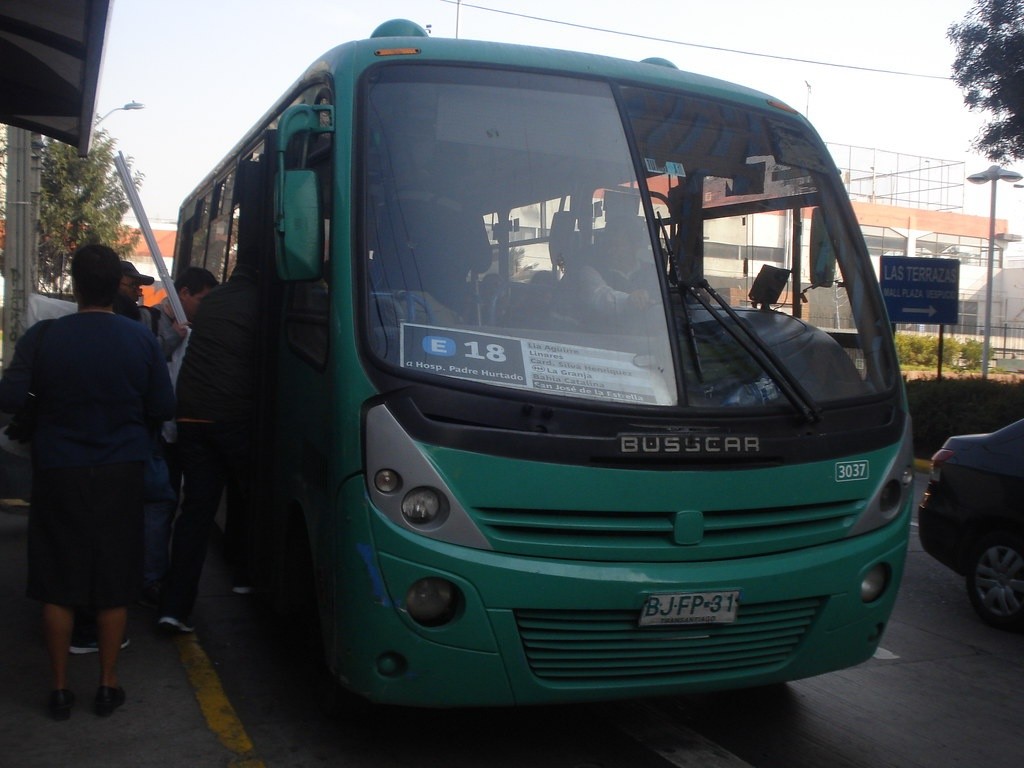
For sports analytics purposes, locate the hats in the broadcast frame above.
[118,261,154,285]
[238,259,274,288]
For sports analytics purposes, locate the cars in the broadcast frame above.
[915,420,1023,633]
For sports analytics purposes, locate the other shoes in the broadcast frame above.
[68,633,130,654]
[51,689,76,717]
[145,577,177,605]
[95,685,125,713]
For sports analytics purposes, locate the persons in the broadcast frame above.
[0,246,176,719]
[386,183,549,329]
[562,217,712,326]
[66,259,281,656]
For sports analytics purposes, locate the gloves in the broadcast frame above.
[3,411,37,443]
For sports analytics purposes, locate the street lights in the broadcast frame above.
[966,166,1024,383]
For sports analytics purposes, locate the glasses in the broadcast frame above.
[119,281,143,294]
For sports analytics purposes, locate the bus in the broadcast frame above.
[169,17,916,717]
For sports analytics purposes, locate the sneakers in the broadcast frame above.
[232,570,255,594]
[157,606,198,632]
